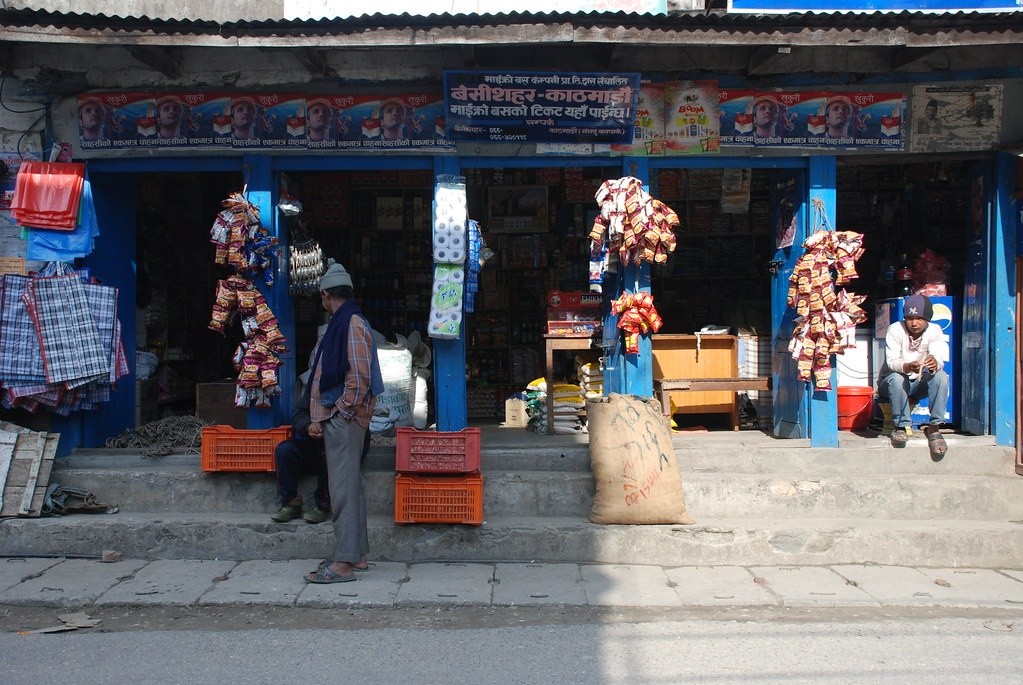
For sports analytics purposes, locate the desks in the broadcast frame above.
[545,338,603,436]
[654,376,772,438]
[651,334,739,432]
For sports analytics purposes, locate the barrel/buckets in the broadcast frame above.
[837,386,874,430]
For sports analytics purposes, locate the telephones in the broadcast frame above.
[699,325,731,335]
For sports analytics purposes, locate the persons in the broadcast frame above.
[142,95,185,145]
[499,189,519,217]
[76,96,108,145]
[877,294,951,456]
[271,392,372,524]
[821,95,851,140]
[303,263,385,583]
[750,96,779,139]
[379,97,406,141]
[917,98,943,135]
[227,96,258,141]
[306,98,331,142]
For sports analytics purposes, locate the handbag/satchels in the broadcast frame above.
[0,261,131,416]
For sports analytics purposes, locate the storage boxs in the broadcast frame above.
[201,424,295,473]
[395,425,483,525]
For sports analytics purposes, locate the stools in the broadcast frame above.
[876,398,919,436]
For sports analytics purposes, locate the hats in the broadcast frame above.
[379,98,405,116]
[305,99,332,118]
[902,295,933,323]
[319,259,354,292]
[156,95,182,112]
[78,96,104,114]
[231,96,256,115]
[753,95,778,115]
[825,96,852,114]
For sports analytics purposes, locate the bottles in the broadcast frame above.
[899,253,913,295]
[513,320,539,345]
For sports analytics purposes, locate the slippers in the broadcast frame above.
[924,427,948,455]
[318,556,369,571]
[302,565,358,583]
[891,428,909,443]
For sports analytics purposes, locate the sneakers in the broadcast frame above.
[303,504,329,523]
[271,494,303,520]
